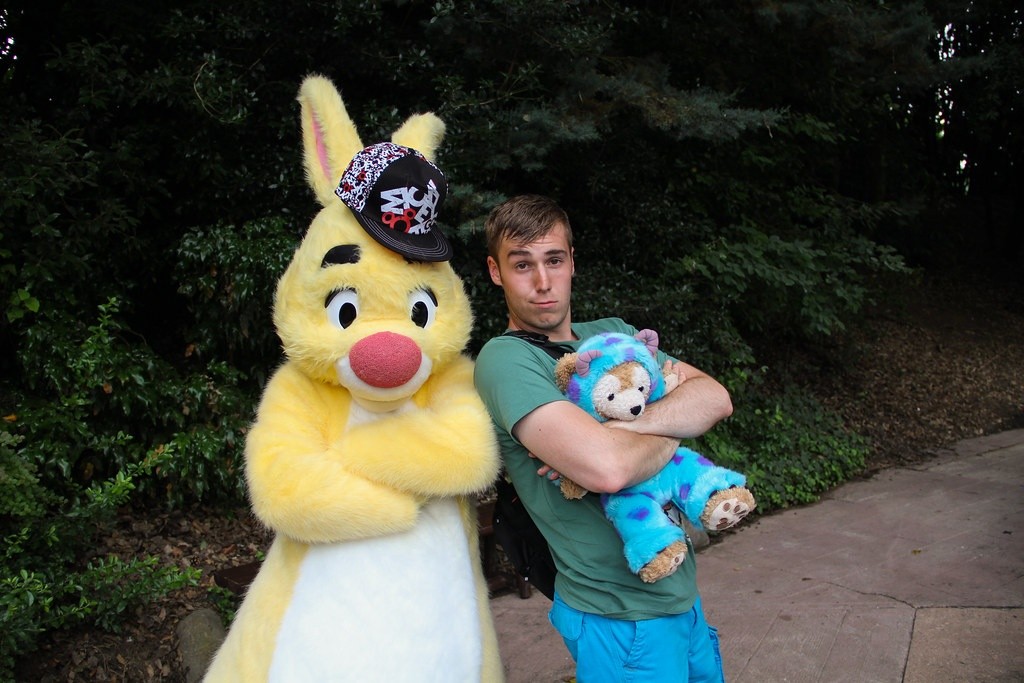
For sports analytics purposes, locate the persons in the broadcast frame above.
[473,196,734,683]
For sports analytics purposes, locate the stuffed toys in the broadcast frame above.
[197,77,503,683]
[543,330,756,584]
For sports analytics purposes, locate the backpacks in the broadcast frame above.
[488,330,577,601]
